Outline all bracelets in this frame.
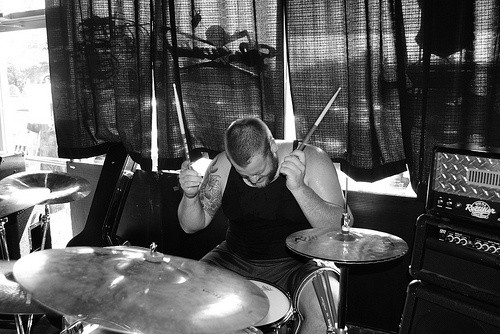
[184,192,198,199]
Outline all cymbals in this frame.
[0,259,42,314]
[285,227,409,265]
[0,187,50,218]
[11,246,269,334]
[0,170,92,204]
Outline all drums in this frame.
[245,276,295,330]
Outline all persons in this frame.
[178,118,354,334]
